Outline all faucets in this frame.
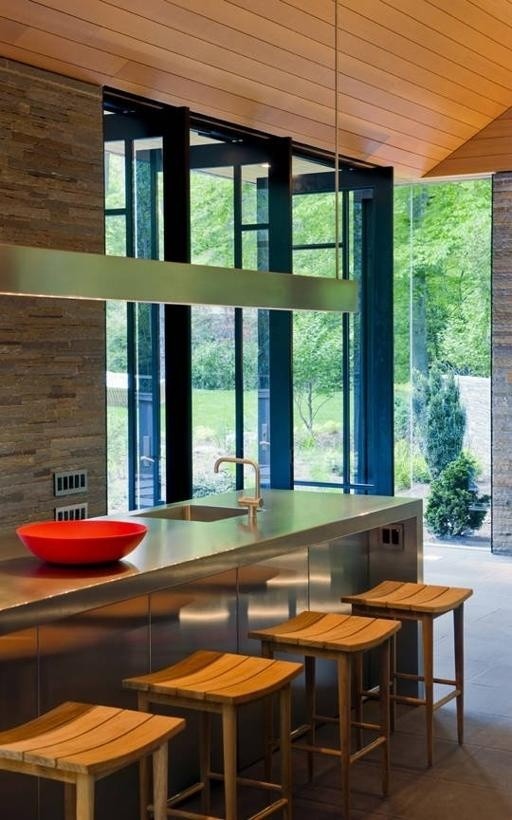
[213,455,263,518]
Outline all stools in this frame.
[0,701,187,820]
[122,649,305,820]
[340,580,472,767]
[247,611,393,820]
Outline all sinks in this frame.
[127,500,269,524]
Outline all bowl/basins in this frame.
[14,519,150,565]
